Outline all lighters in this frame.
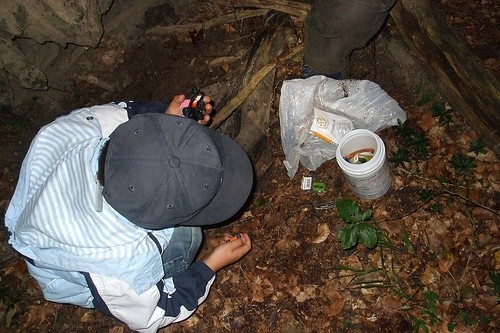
[230,234,242,241]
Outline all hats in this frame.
[103,112,253,230]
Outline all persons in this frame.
[302,0,396,79]
[4,95,251,333]
